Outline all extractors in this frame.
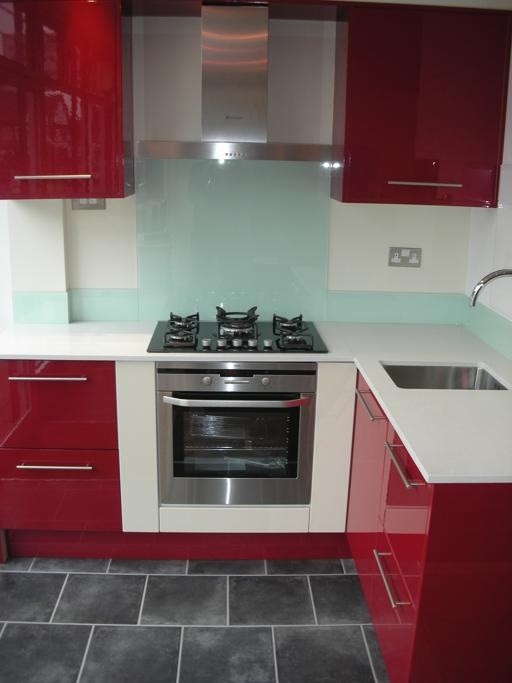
[138,4,347,165]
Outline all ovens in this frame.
[153,361,320,508]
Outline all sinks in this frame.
[377,359,512,390]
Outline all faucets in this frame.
[469,269,512,308]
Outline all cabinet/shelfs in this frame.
[0,360,122,533]
[368,421,512,683]
[331,1,512,207]
[0,0,135,199]
[345,371,389,607]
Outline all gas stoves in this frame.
[146,303,333,353]
